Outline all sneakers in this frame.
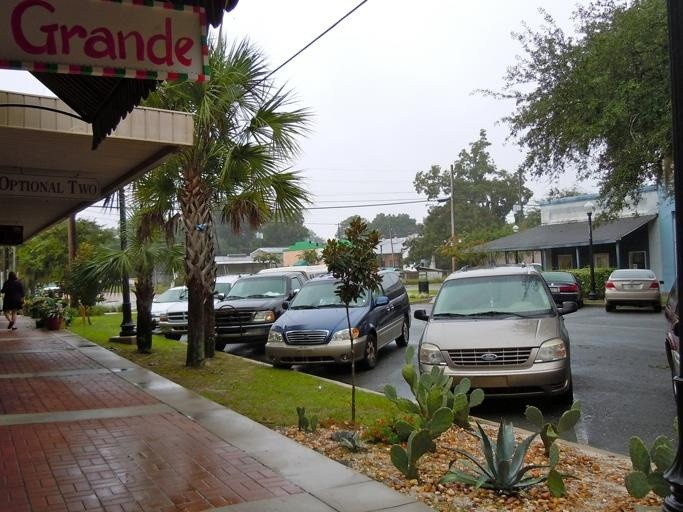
[8,321,17,330]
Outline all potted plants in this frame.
[42,300,64,330]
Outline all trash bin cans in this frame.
[419,282,428,294]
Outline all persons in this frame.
[0,271,25,329]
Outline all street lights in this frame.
[437,165,457,272]
[513,225,519,264]
[584,201,598,300]
